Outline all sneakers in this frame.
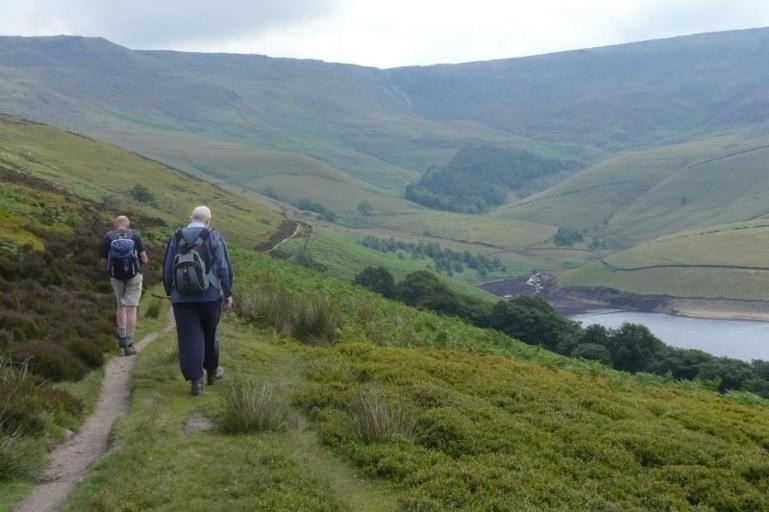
[207,367,224,384]
[190,379,203,395]
[119,337,137,356]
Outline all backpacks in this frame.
[171,227,213,294]
[107,231,139,281]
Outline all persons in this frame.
[99,215,149,356]
[162,205,234,396]
